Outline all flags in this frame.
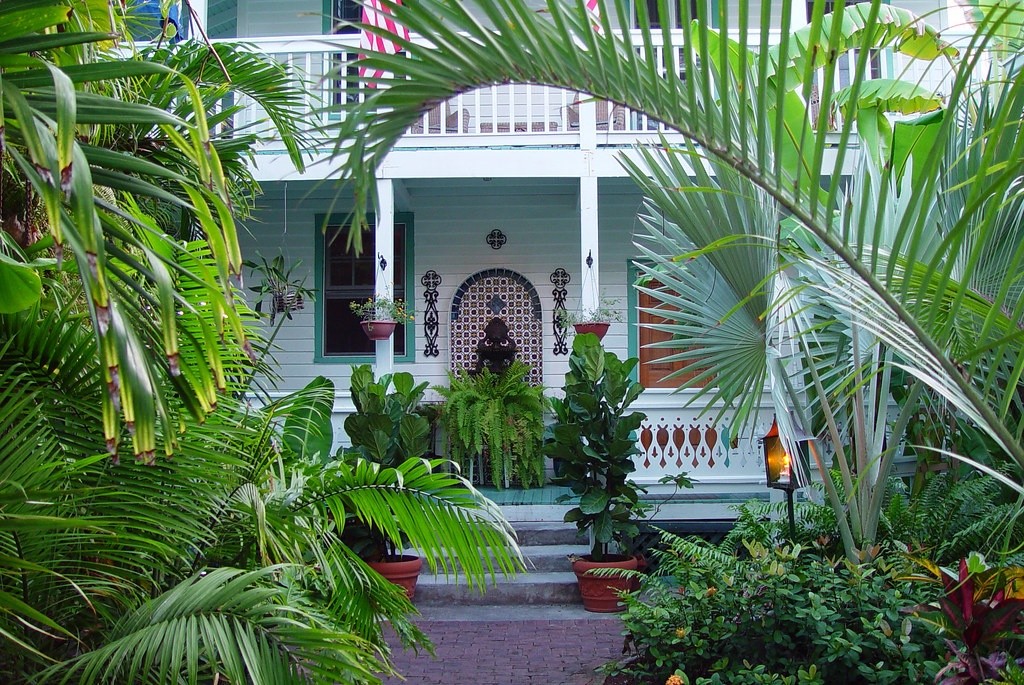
[587,0,602,35]
[157,0,184,50]
[358,0,410,88]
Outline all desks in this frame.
[480,122,558,133]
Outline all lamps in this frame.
[757,411,819,546]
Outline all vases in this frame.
[359,321,398,341]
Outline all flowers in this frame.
[349,281,420,332]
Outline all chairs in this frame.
[412,101,470,134]
[810,85,836,131]
[560,91,625,131]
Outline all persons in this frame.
[477,318,516,375]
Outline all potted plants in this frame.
[241,247,320,326]
[553,286,627,341]
[337,364,433,603]
[537,332,700,615]
